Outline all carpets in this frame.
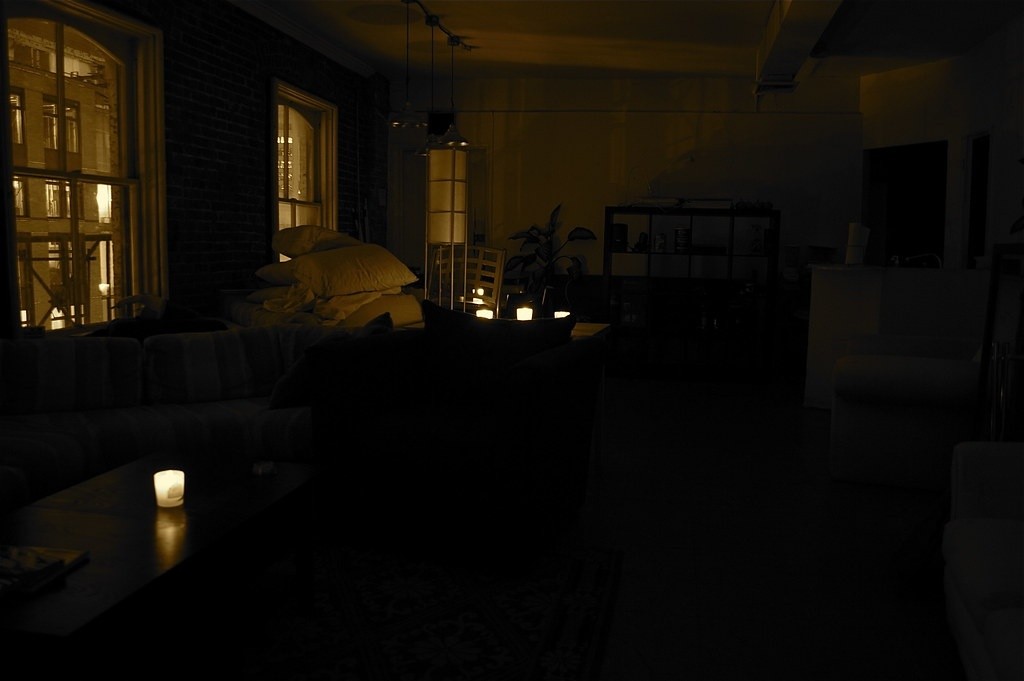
[225,537,624,681]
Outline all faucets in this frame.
[904,252,944,271]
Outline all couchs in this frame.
[303,329,609,579]
[829,331,982,489]
[0,322,312,513]
[942,440,1024,681]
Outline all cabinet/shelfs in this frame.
[803,264,1024,410]
[602,206,781,387]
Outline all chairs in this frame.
[427,245,507,319]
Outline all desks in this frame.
[0,447,317,681]
[571,322,612,479]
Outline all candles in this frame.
[517,307,533,320]
[476,309,493,319]
[554,311,570,318]
[153,469,185,508]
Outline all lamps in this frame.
[386,0,429,129]
[424,147,469,313]
[414,14,450,157]
[435,34,469,147]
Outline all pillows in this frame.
[266,330,353,411]
[420,300,578,414]
[0,337,142,415]
[285,244,419,299]
[272,225,365,258]
[143,324,290,404]
[246,286,288,304]
[254,262,299,286]
[354,313,394,338]
[336,293,423,327]
